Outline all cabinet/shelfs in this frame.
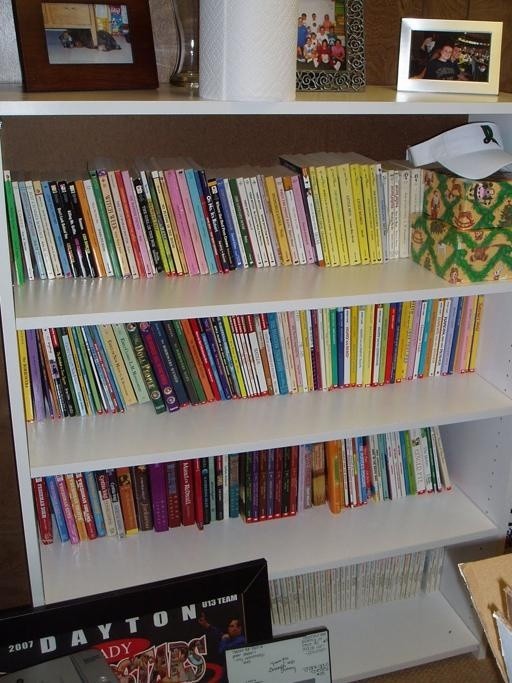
[0,0,510,681]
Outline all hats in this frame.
[404,122,511,181]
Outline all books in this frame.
[18,294,485,425]
[32,422,453,546]
[267,544,448,624]
[4,152,426,286]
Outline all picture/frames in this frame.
[10,0,161,94]
[395,19,503,96]
[295,0,367,93]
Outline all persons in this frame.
[298,12,345,70]
[196,612,245,658]
[409,36,489,82]
